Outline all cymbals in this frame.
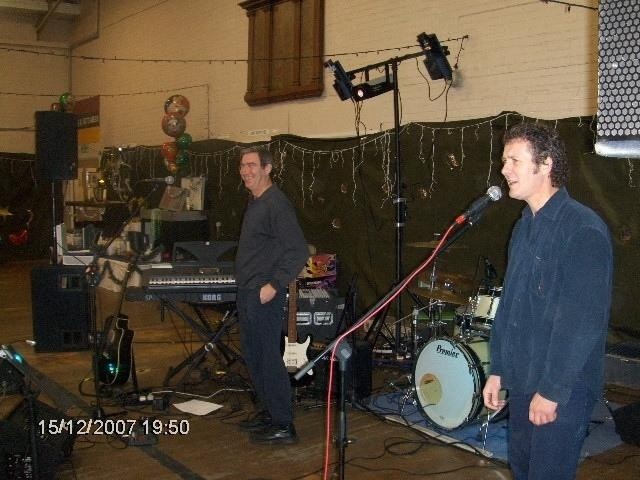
[404,241,469,250]
[406,269,479,306]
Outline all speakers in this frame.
[30,264,96,353]
[34,110,78,181]
[0,397,90,480]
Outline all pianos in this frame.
[64,199,127,208]
[141,261,289,303]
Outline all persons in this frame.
[231,144,311,445]
[481,122,613,480]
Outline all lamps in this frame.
[321,32,459,352]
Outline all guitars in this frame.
[92,246,145,387]
[281,278,314,388]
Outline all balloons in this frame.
[161,94,192,183]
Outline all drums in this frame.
[453,285,504,338]
[410,331,508,432]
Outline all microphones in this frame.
[457,186,503,225]
[138,176,175,185]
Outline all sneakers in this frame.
[239,410,273,432]
[249,421,297,442]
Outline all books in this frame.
[179,176,207,211]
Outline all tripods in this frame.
[346,51,448,354]
[72,185,162,446]
